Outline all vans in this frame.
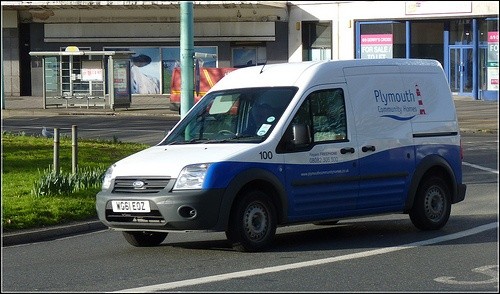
[94,58,467,252]
[168,67,238,114]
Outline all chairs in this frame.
[291,124,312,145]
[237,92,273,138]
